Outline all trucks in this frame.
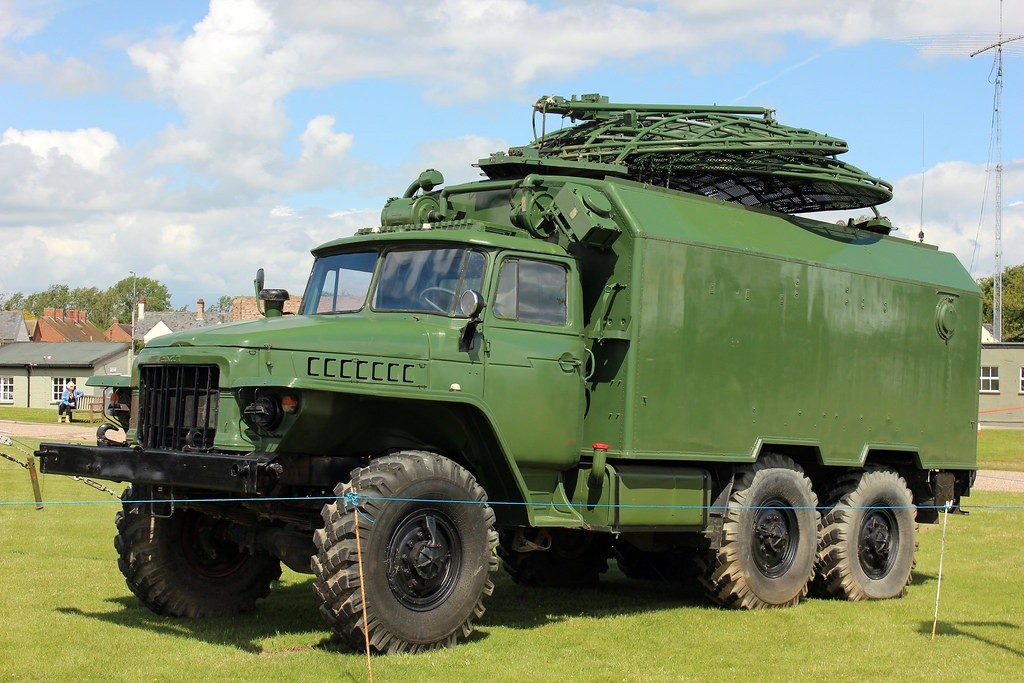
[32,95,985,655]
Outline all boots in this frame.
[65,415,70,424]
[58,415,62,423]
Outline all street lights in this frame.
[130,271,136,367]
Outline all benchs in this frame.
[51,395,105,424]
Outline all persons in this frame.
[57,381,84,423]
